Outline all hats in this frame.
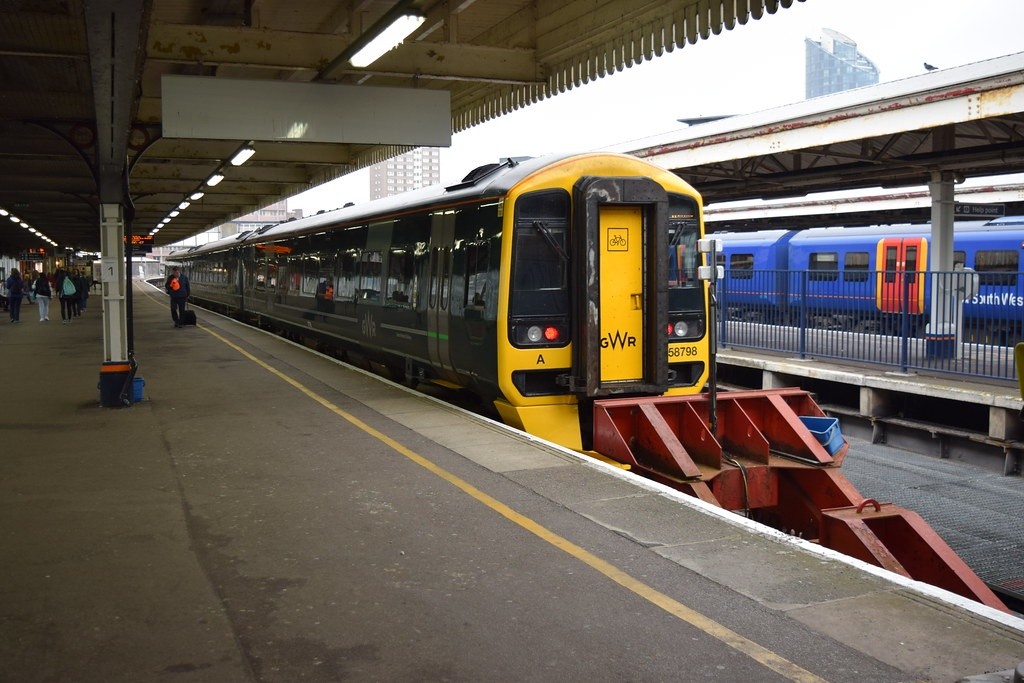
[172,266,179,271]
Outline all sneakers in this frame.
[40,318,45,322]
[45,316,50,321]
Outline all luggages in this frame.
[182,299,196,325]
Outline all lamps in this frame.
[149,189,206,236]
[230,146,256,166]
[206,170,225,187]
[0,208,59,247]
[347,7,428,67]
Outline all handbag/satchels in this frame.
[31,280,37,290]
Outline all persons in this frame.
[314,273,338,313]
[0,268,90,324]
[165,266,190,328]
[6,268,23,323]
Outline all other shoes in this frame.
[179,324,183,328]
[68,319,72,323]
[13,320,22,323]
[175,319,179,327]
[10,318,15,322]
[63,320,67,325]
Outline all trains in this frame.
[164,151,725,469]
[664,215,1024,347]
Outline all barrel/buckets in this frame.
[925,322,956,359]
[100,361,132,407]
[133,376,145,401]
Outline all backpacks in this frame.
[10,277,21,293]
[63,275,77,296]
